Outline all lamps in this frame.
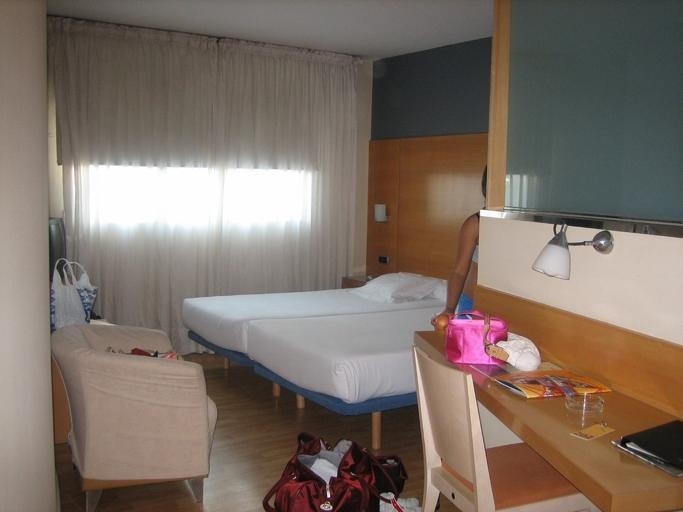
[531,218,614,281]
[373,203,387,223]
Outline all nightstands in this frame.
[341,271,387,289]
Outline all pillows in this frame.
[347,272,444,304]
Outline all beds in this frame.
[183,289,446,371]
[245,307,447,450]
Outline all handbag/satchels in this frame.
[446,311,507,368]
[260,431,409,511]
[483,314,541,373]
[49,256,99,330]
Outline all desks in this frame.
[413,329,683,512]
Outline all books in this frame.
[619,418,683,471]
[468,359,566,382]
[609,435,682,479]
[492,368,612,398]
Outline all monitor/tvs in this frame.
[49,216,68,282]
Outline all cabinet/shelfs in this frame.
[50,318,111,444]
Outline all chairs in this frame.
[50,324,218,512]
[411,344,593,512]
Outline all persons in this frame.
[430,165,487,331]
